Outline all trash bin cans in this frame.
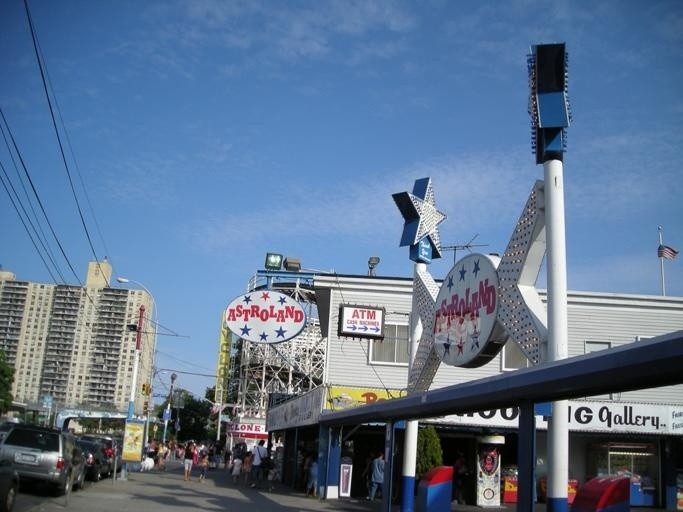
[570,475,630,512]
[418,466,454,512]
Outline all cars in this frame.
[0,421,122,495]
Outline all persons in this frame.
[368,451,386,503]
[141,435,320,498]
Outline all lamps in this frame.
[264,253,301,272]
[366,256,380,276]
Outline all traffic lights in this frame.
[142,384,146,396]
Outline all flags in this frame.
[657,245,679,262]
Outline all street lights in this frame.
[117,276,158,413]
[162,371,181,438]
